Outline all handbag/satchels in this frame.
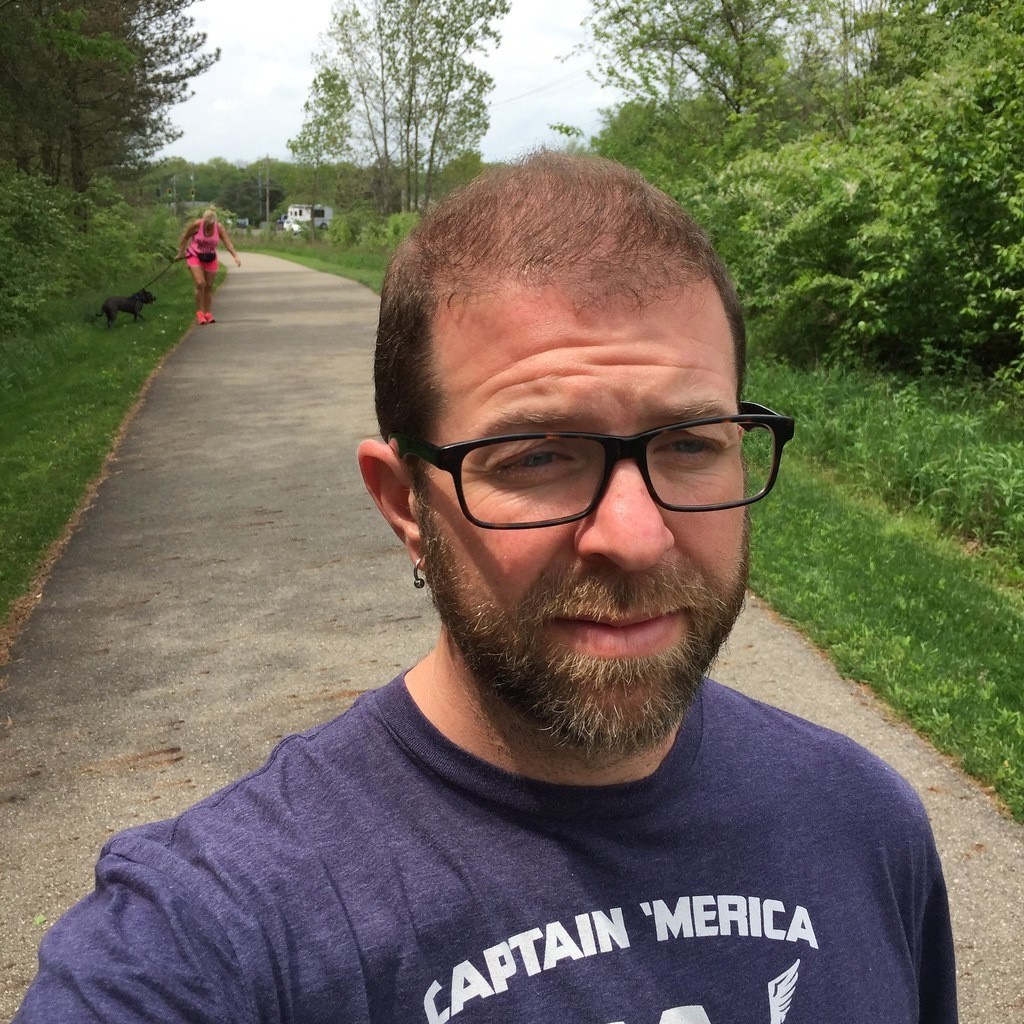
[190,246,216,262]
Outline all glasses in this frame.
[387,402,795,530]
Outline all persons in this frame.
[14,152,958,1024]
[175,206,241,325]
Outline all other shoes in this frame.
[196,311,207,325]
[204,312,215,323]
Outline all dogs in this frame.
[95,287,155,329]
